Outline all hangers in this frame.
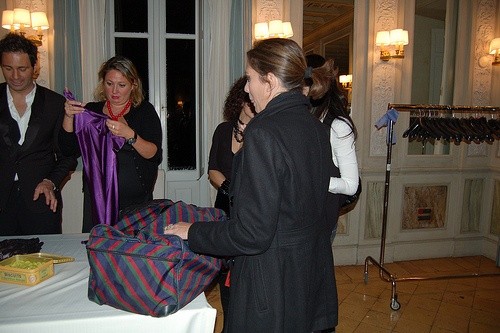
[402,104,500,146]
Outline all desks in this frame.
[0,233,218,333]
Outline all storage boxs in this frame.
[0,252,74,287]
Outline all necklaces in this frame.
[107,100,132,121]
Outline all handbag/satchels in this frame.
[83,198,232,317]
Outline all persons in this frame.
[60,55,161,235]
[162,38,362,333]
[206,75,256,216]
[301,51,359,197]
[0,34,81,235]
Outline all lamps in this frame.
[488,37,500,66]
[254,19,294,41]
[375,29,409,61]
[338,73,352,88]
[1,8,50,47]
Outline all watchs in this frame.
[127,131,139,146]
